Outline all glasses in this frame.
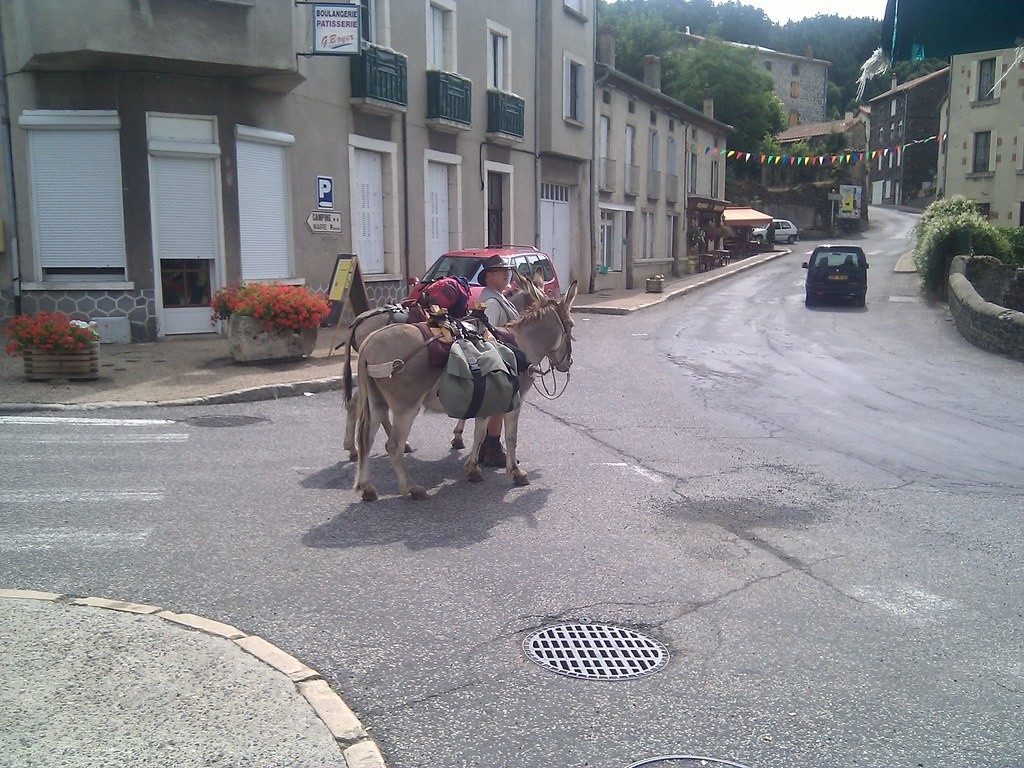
[486,266,512,275]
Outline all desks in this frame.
[698,254,713,270]
[716,250,729,265]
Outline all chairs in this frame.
[698,249,730,273]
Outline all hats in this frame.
[477,254,516,286]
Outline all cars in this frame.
[750,219,800,244]
[408,245,562,329]
[801,244,869,308]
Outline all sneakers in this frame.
[479,443,487,463]
[483,443,520,467]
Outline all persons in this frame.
[766,219,775,251]
[937,187,943,200]
[476,255,521,468]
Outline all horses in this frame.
[341,266,579,502]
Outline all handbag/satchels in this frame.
[439,339,522,419]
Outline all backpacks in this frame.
[417,276,471,317]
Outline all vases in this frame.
[23,344,99,380]
[222,315,318,364]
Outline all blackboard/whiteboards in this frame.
[319,252,372,325]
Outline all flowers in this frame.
[208,279,333,338]
[4,314,99,355]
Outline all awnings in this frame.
[722,207,774,227]
